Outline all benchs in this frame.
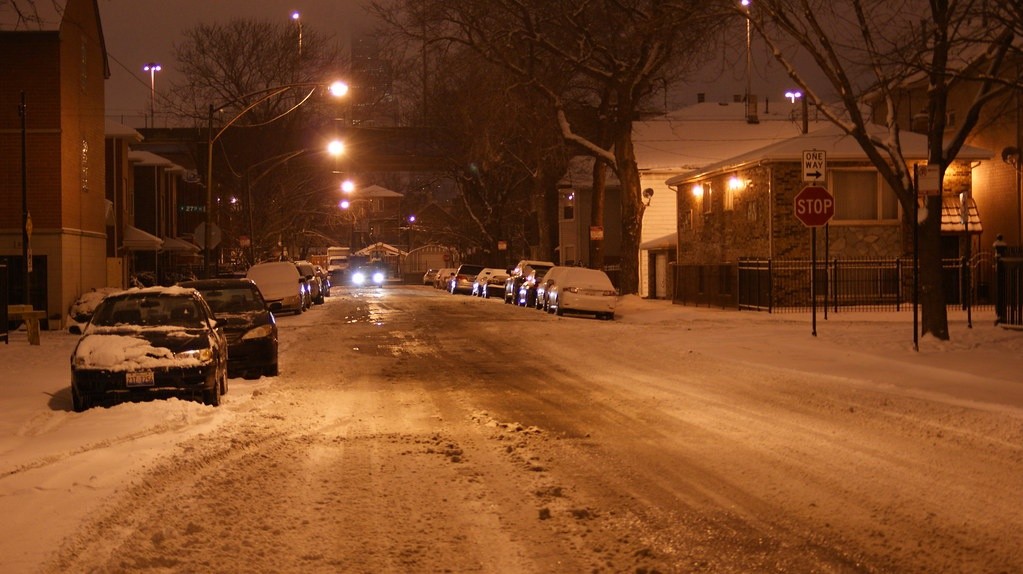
[7,311,47,345]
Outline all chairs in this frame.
[114,308,143,326]
[170,304,196,321]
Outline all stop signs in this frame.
[794,185,836,229]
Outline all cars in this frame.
[356,265,383,288]
[423,260,617,320]
[168,279,283,378]
[67,285,229,412]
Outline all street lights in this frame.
[144,61,162,127]
[292,9,306,93]
[245,139,345,266]
[203,81,349,277]
[282,178,354,257]
[741,0,753,101]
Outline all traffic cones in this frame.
[241,246,352,316]
